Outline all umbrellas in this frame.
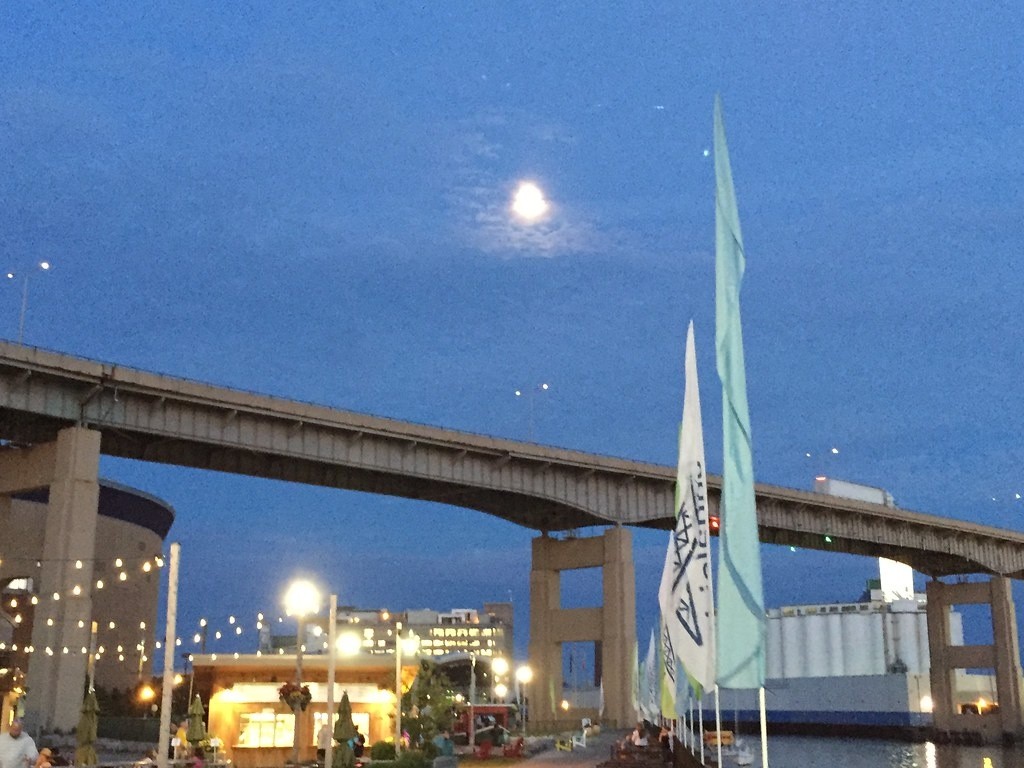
[77,689,99,766]
[332,690,356,768]
[186,695,205,758]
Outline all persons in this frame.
[483,715,490,727]
[623,735,633,759]
[631,723,651,765]
[659,727,680,768]
[317,725,334,761]
[0,723,68,768]
[354,726,365,757]
[403,728,409,751]
[168,722,188,759]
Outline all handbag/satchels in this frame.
[172,738,181,747]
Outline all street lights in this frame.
[516,666,532,737]
[280,578,322,767]
